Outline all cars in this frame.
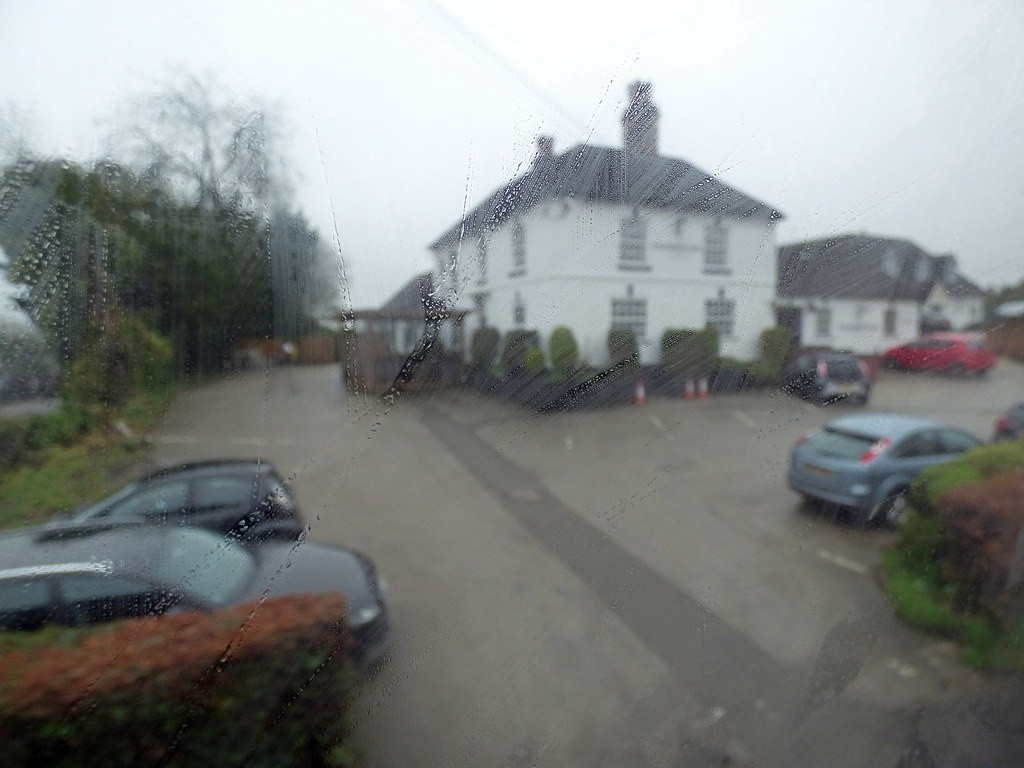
[70,459,307,541]
[782,349,872,404]
[990,401,1024,442]
[880,332,998,376]
[786,412,986,531]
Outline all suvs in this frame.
[0,516,390,670]
